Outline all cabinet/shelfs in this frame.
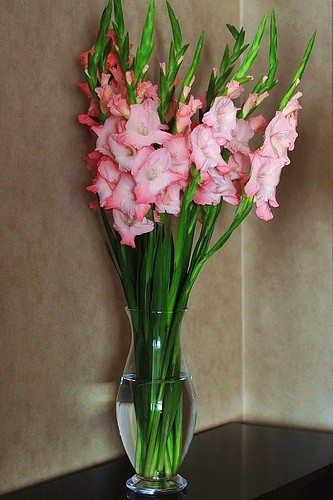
[1,422,332,498]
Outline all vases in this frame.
[115,306,198,494]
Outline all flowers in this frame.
[77,1,317,479]
